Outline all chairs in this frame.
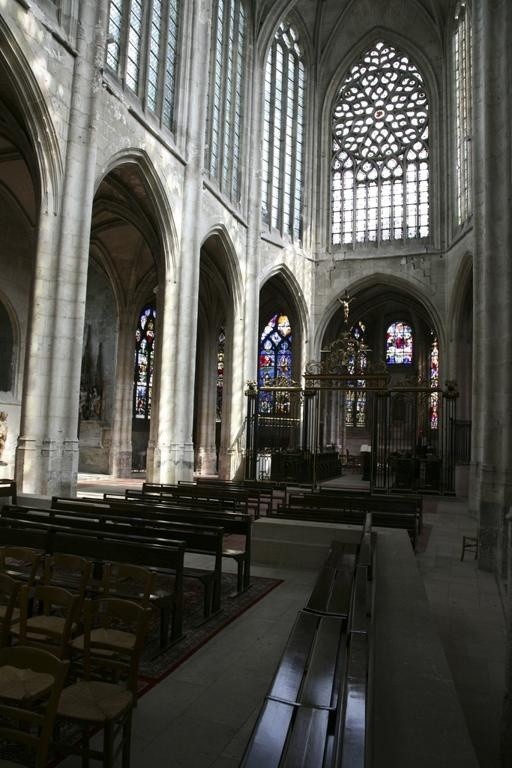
[1,584,151,768]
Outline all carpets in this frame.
[1,555,286,768]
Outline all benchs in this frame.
[0,477,424,660]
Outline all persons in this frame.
[336,296,357,323]
[85,385,103,420]
[0,410,10,467]
[78,384,90,422]
